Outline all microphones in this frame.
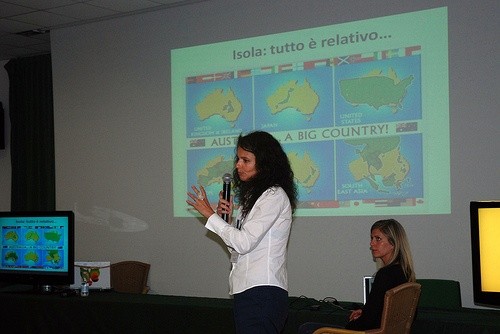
[222,173,231,223]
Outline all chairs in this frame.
[312,282,422,334]
[416,279,462,307]
[109,261,152,294]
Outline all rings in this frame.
[200,197,205,200]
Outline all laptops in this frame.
[363,277,377,306]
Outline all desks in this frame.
[0,286,500,334]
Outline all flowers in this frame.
[80,266,100,285]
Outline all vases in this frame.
[81,284,89,296]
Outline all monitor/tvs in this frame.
[470,201,500,309]
[0,211,74,296]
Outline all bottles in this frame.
[81,278,89,296]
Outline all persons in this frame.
[185,132,298,334]
[297,218,416,334]
